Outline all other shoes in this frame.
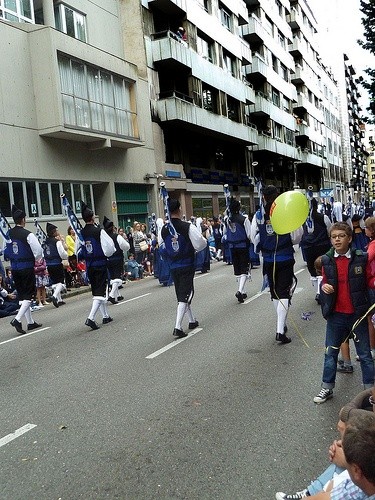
[235,291,247,303]
[85,318,100,330]
[108,295,123,304]
[102,316,113,324]
[50,296,59,308]
[27,321,43,331]
[276,333,292,343]
[58,301,66,306]
[173,328,188,337]
[30,301,50,312]
[336,360,354,373]
[10,318,26,334]
[189,321,199,329]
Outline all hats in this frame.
[46,222,58,232]
[81,201,94,219]
[12,204,25,221]
[167,194,181,211]
[103,216,113,229]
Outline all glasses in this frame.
[331,233,348,240]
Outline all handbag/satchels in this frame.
[139,240,149,252]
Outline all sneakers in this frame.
[275,489,310,500]
[314,388,333,403]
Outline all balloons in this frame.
[270,191,309,235]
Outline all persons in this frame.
[319,200,374,222]
[313,217,375,402]
[66,226,77,287]
[251,184,303,342]
[75,202,116,329]
[276,385,375,500]
[224,200,251,303]
[3,205,42,334]
[102,217,130,303]
[160,197,207,338]
[0,267,20,318]
[113,220,158,284]
[41,223,69,308]
[34,258,49,308]
[299,198,332,303]
[181,215,224,261]
